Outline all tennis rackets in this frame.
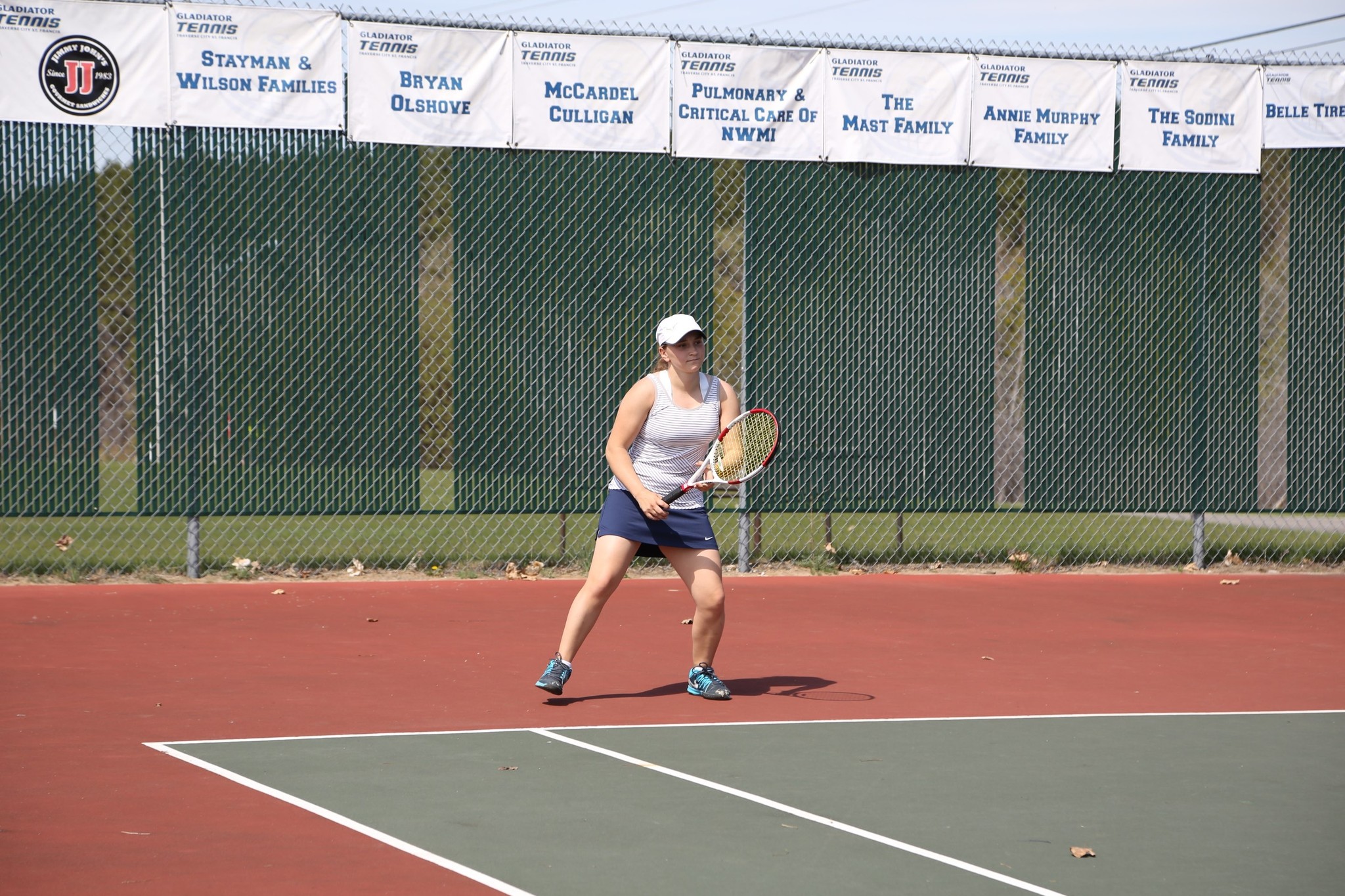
[659,407,782,510]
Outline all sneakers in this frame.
[687,662,731,699]
[535,652,572,695]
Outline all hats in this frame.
[655,314,707,347]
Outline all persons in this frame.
[535,313,745,698]
